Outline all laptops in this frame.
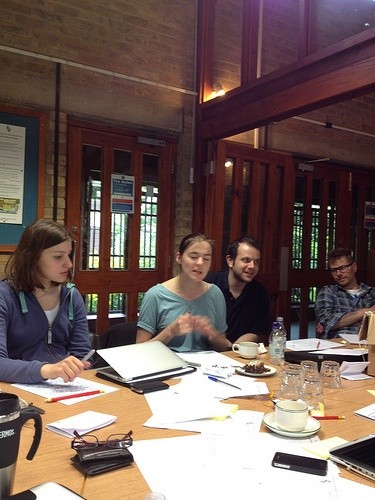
[96,340,188,382]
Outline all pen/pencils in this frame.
[314,416,345,420]
[317,342,321,348]
[45,390,105,403]
[208,377,241,390]
[186,361,202,367]
[82,350,95,362]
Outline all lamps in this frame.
[212,82,225,97]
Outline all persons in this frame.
[135,232,228,352]
[0,218,94,383]
[203,236,271,345]
[315,248,375,340]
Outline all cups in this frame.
[0,392,43,500]
[320,361,341,389]
[273,399,309,432]
[232,342,259,359]
[279,360,324,409]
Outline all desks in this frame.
[0,337,375,500]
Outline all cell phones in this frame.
[272,452,328,476]
[131,381,169,394]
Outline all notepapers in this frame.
[299,436,349,460]
[204,403,239,421]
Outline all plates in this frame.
[235,365,277,377]
[262,412,321,437]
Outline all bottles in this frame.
[268,317,287,365]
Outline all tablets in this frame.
[328,435,375,479]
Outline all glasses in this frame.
[329,262,354,275]
[71,430,133,450]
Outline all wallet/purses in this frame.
[72,439,134,475]
